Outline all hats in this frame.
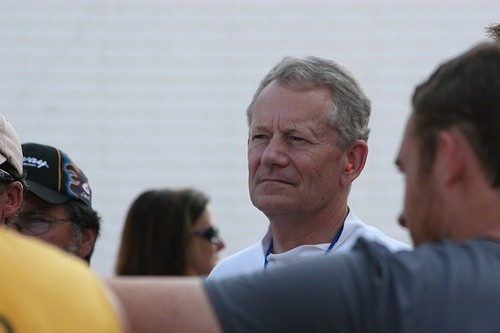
[20,142,92,207]
[0,114,23,175]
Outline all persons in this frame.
[207,56,412,281]
[0,114,123,333]
[100,40,500,333]
[5,142,99,267]
[115,188,226,278]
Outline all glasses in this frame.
[19,211,71,236]
[192,226,219,244]
